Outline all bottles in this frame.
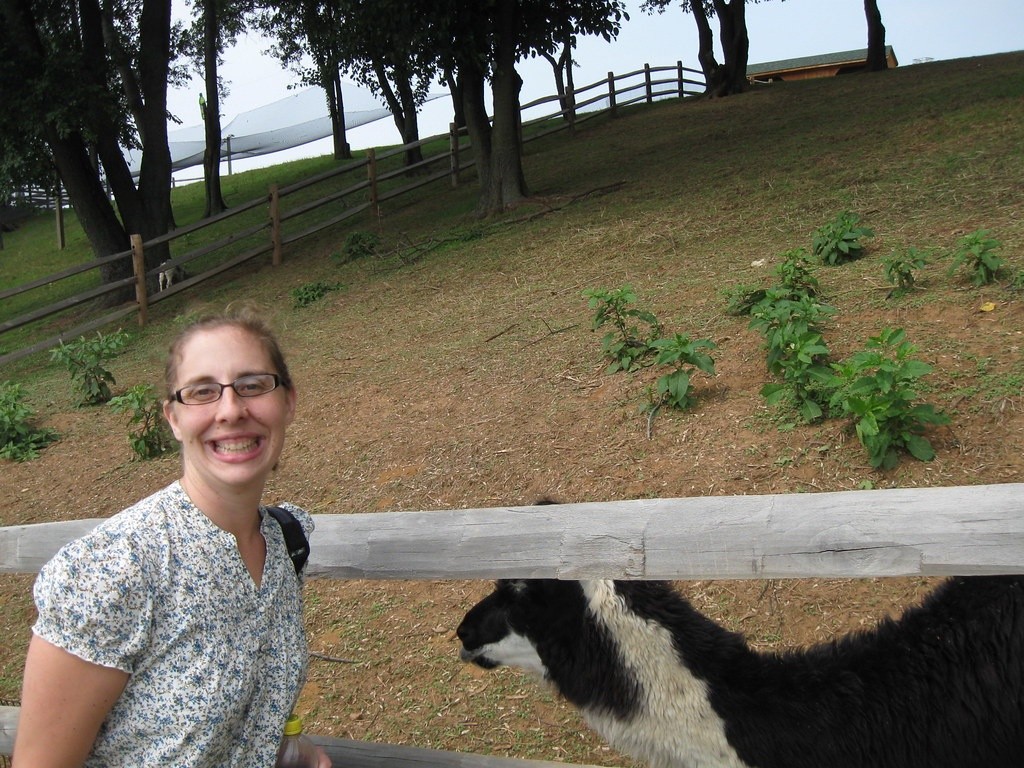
[275,714,319,768]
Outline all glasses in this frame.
[169,373,291,405]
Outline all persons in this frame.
[11,312,335,768]
